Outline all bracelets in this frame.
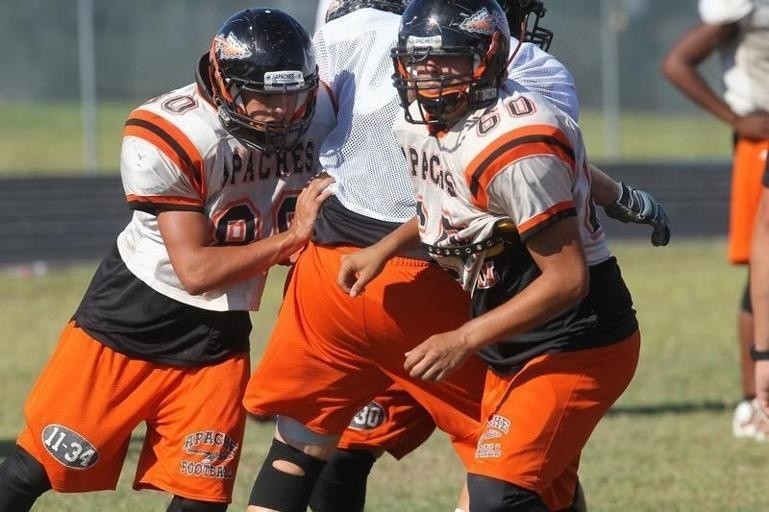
[749,342,769,362]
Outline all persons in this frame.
[2,7,335,511]
[745,144,769,425]
[242,0,673,510]
[658,0,769,440]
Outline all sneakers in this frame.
[733,400,768,443]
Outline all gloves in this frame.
[609,183,670,247]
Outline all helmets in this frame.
[327,1,552,125]
[210,7,319,154]
